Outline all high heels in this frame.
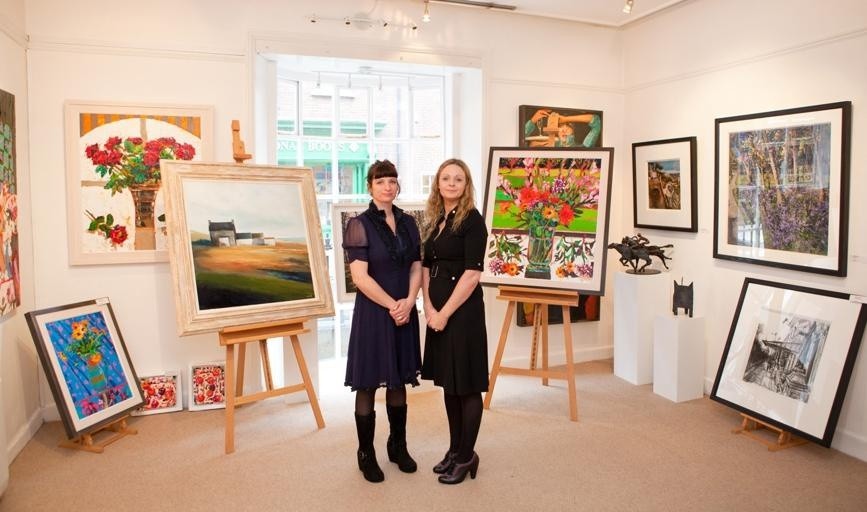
[438,449,479,484]
[432,448,459,473]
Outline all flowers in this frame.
[83,208,128,248]
[58,320,107,366]
[497,157,600,264]
[86,136,196,227]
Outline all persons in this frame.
[342,159,422,482]
[420,158,489,484]
[524,107,600,149]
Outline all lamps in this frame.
[422,1,430,22]
[622,0,633,15]
[309,14,417,30]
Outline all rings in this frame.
[398,316,402,320]
[434,329,438,332]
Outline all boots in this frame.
[386,404,417,473]
[355,410,384,483]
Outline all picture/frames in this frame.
[331,203,430,303]
[160,159,336,338]
[518,105,603,327]
[24,297,146,440]
[710,277,866,448]
[131,369,183,417]
[632,136,698,233]
[478,147,614,296]
[64,102,217,266]
[712,100,850,278]
[188,361,226,411]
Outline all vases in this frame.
[86,360,107,391]
[526,224,554,280]
[129,183,160,250]
